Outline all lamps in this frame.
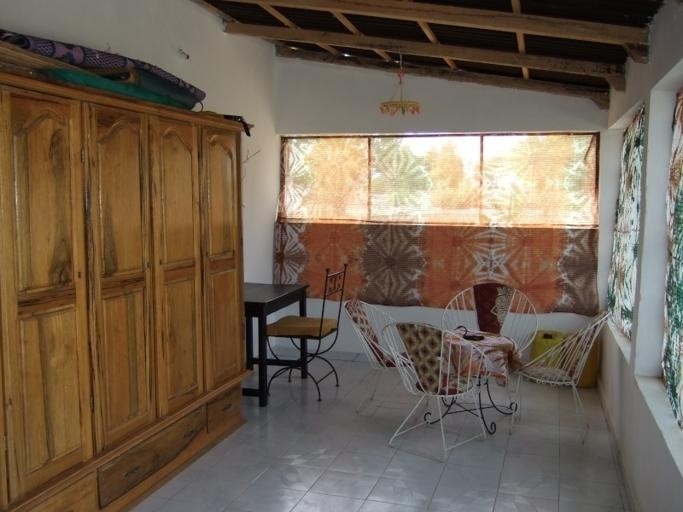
[380,52,421,115]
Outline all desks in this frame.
[243,284,309,407]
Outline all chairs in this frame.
[267,263,348,401]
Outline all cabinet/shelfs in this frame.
[0,73,254,512]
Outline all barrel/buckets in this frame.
[565,342,598,387]
[531,330,562,382]
